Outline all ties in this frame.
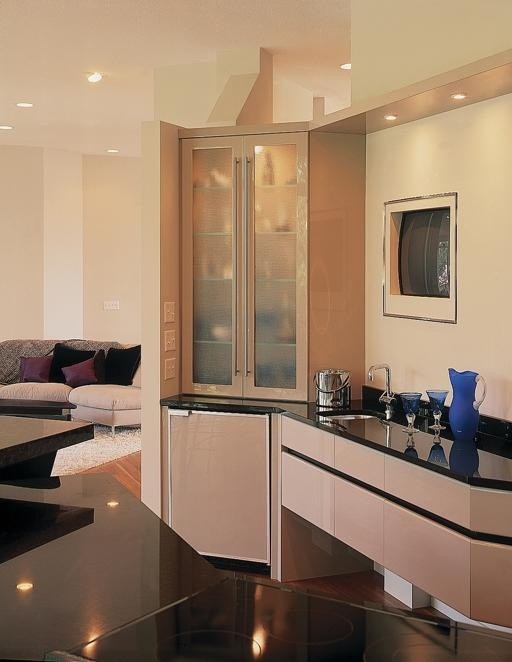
[383,195,456,323]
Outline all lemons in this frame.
[447,367,487,438]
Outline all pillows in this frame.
[18,341,141,387]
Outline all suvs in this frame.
[313,368,352,407]
[316,407,348,432]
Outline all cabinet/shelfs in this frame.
[166,408,272,564]
[280,412,362,556]
[178,131,312,404]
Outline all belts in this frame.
[426,430,449,468]
[399,392,422,433]
[426,390,448,430]
[402,432,420,460]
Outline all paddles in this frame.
[51,417,142,476]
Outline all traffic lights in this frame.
[18,341,141,387]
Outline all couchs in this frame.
[0,339,142,439]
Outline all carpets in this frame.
[51,417,142,476]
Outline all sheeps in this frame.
[385,410,395,449]
[368,362,397,410]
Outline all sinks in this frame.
[0,339,142,439]
